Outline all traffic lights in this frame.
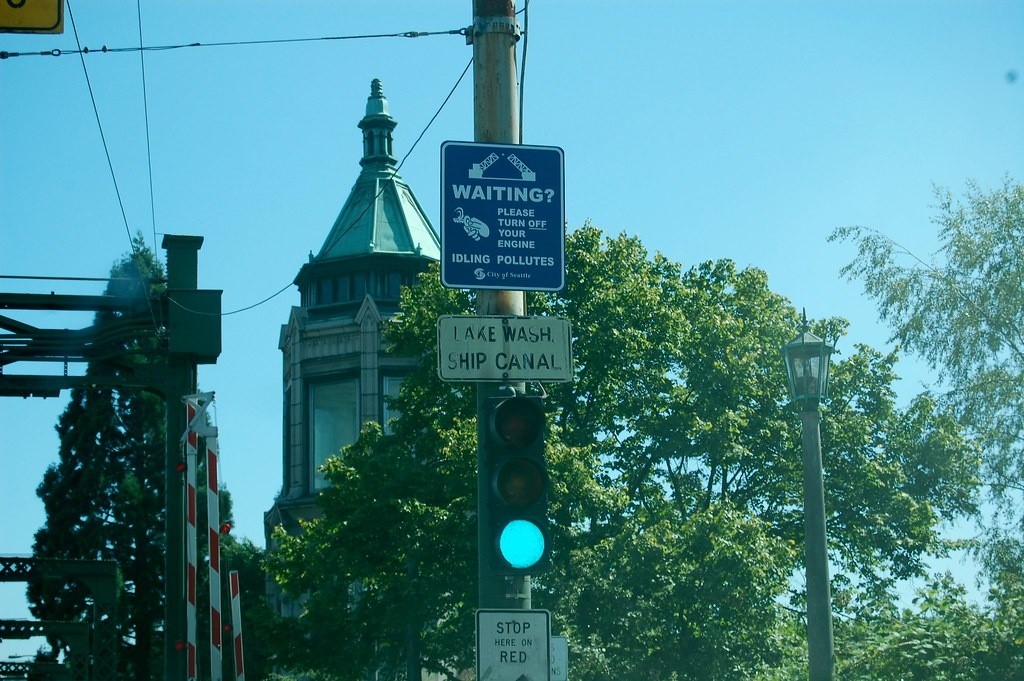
[489,396,551,571]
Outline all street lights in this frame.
[781,307,840,681]
